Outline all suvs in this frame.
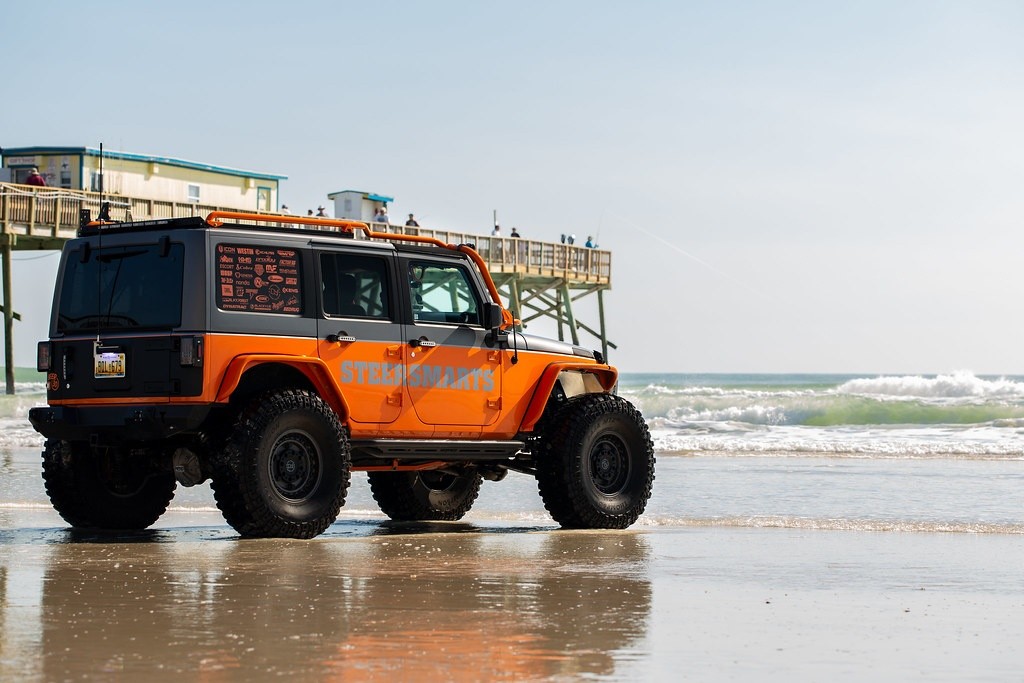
[28,144,656,539]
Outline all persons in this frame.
[305,208,315,230]
[510,227,521,260]
[279,204,291,227]
[316,205,331,231]
[491,224,504,261]
[24,166,46,187]
[585,235,598,273]
[405,213,419,235]
[372,205,393,241]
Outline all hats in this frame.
[28,168,39,175]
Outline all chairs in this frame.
[324,272,366,315]
